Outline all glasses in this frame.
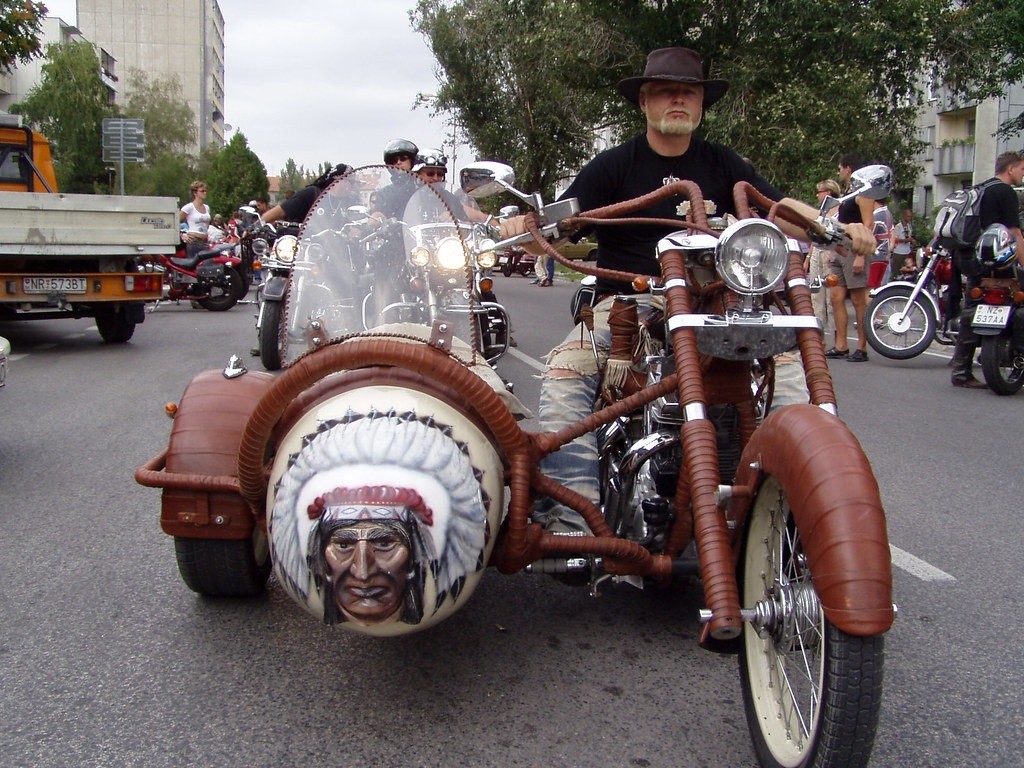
[197,190,207,192]
[392,156,410,164]
[816,190,826,194]
[837,167,845,172]
[423,155,447,165]
[421,170,444,177]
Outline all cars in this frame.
[555,239,598,261]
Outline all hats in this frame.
[617,48,730,109]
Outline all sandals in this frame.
[824,346,850,359]
[847,349,869,362]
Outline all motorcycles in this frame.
[862,187,1024,396]
[133,161,895,768]
[137,182,519,371]
[499,248,534,277]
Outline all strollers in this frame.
[891,244,928,284]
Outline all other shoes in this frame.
[530,279,554,287]
[250,347,260,355]
[954,378,987,389]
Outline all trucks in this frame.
[0,113,180,344]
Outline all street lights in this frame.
[421,94,455,181]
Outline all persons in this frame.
[499,47,877,587]
[803,155,874,360]
[241,163,353,356]
[873,197,918,306]
[348,139,518,347]
[532,253,553,288]
[180,180,296,309]
[951,152,1024,389]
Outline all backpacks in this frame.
[934,180,1003,248]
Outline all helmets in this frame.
[412,148,448,173]
[383,139,418,161]
[976,223,1017,270]
